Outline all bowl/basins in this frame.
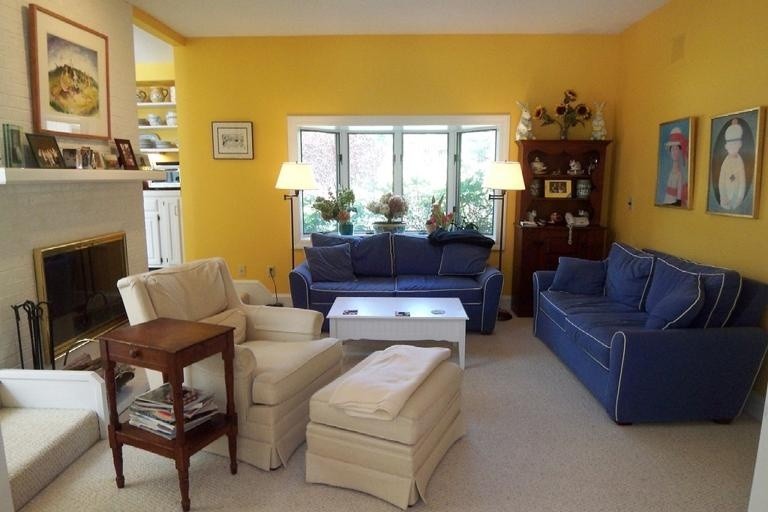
[148,114,161,125]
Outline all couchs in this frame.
[290,228,503,337]
[533,243,767,426]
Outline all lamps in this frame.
[480,160,526,322]
[274,161,320,272]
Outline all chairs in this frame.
[118,256,351,474]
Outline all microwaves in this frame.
[146,165,180,188]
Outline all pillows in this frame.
[642,273,706,331]
[647,257,726,325]
[604,240,653,312]
[301,244,357,282]
[437,227,494,277]
[549,251,605,300]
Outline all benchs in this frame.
[0,363,111,510]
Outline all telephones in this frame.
[565,212,590,228]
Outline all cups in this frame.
[166,111,177,124]
[169,87,175,102]
[82,150,96,170]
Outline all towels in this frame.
[330,342,455,420]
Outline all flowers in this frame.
[427,194,456,233]
[536,86,592,130]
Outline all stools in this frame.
[303,350,471,507]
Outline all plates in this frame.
[139,138,153,148]
[138,133,160,148]
[156,141,170,149]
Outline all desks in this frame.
[100,316,245,510]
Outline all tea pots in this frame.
[148,87,167,102]
[532,157,548,175]
[136,89,146,102]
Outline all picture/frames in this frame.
[114,137,141,169]
[24,132,70,169]
[703,106,767,219]
[542,178,572,198]
[28,3,113,142]
[211,120,255,160]
[654,115,697,211]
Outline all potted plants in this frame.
[315,182,357,235]
[365,192,409,233]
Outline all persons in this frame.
[719,119,746,209]
[38,141,60,167]
[552,182,565,193]
[665,127,688,206]
[12,133,22,164]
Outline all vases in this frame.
[424,221,437,233]
[560,125,569,138]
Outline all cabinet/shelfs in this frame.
[134,101,179,153]
[510,138,614,320]
[142,193,184,268]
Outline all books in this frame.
[129,382,218,439]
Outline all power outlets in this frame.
[265,265,276,277]
